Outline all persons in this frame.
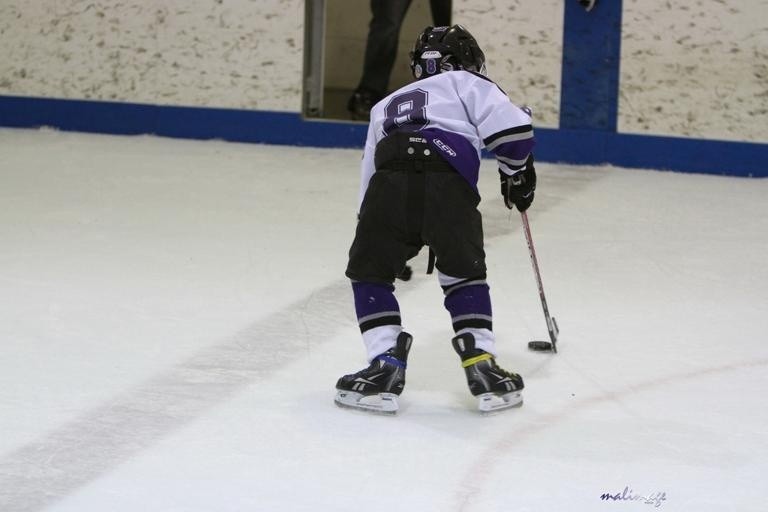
[347,0,452,118]
[335,22,542,397]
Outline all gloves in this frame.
[495,152,537,213]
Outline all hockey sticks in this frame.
[521,211,558,354]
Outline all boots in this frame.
[336,331,413,394]
[451,332,524,396]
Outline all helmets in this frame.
[408,24,486,81]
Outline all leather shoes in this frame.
[350,87,381,122]
